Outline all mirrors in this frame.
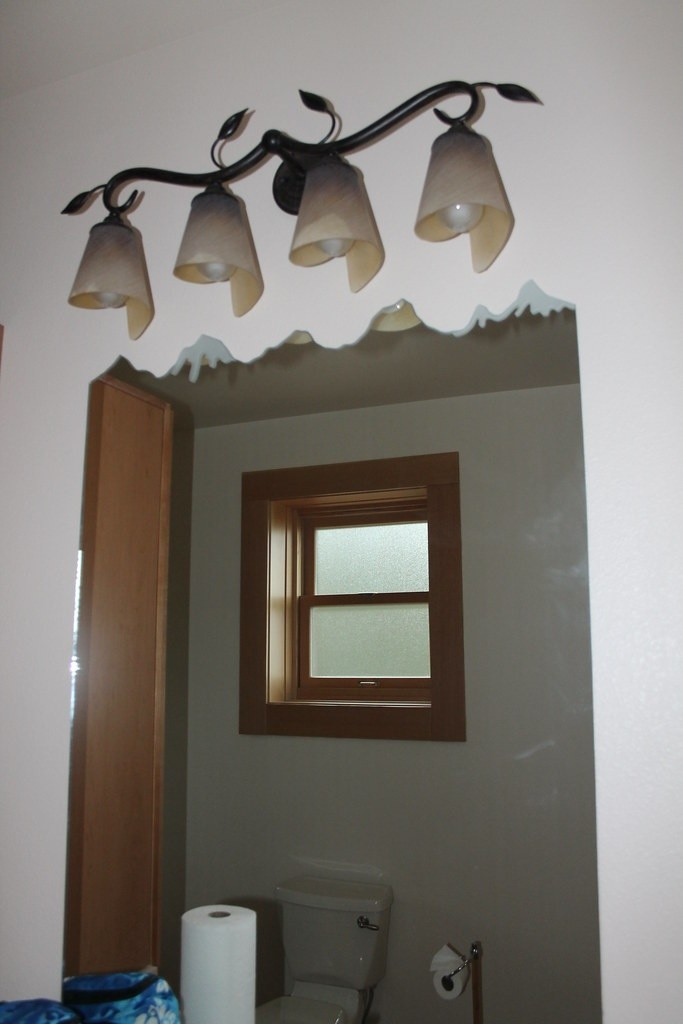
[61,298,606,1024]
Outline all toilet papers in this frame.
[180,904,257,1023]
[429,943,469,1000]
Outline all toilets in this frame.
[258,878,393,1024]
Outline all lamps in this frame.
[56,71,544,341]
